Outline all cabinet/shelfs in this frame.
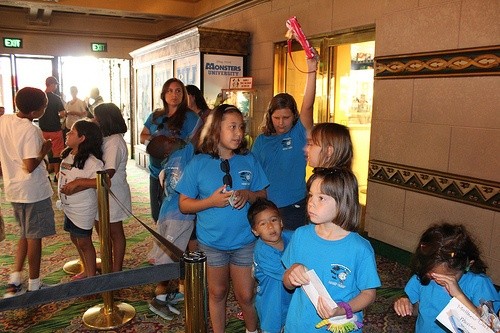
[132,28,253,170]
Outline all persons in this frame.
[247,47,320,232]
[243,122,253,150]
[0,86,55,297]
[247,199,298,333]
[58,119,105,301]
[303,122,353,173]
[58,86,105,148]
[392,222,500,333]
[0,107,4,115]
[140,78,213,321]
[54,104,131,274]
[179,103,268,333]
[283,167,378,333]
[38,77,66,184]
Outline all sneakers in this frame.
[149,292,176,320]
[149,295,180,315]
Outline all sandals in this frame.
[78,294,96,302]
[70,274,84,281]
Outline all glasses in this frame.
[421,245,466,259]
[312,167,344,181]
[221,159,232,189]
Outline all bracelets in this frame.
[338,301,353,319]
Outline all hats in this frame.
[46,77,60,85]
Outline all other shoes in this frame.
[4,284,24,298]
[173,293,184,301]
[54,176,58,182]
[25,284,48,292]
